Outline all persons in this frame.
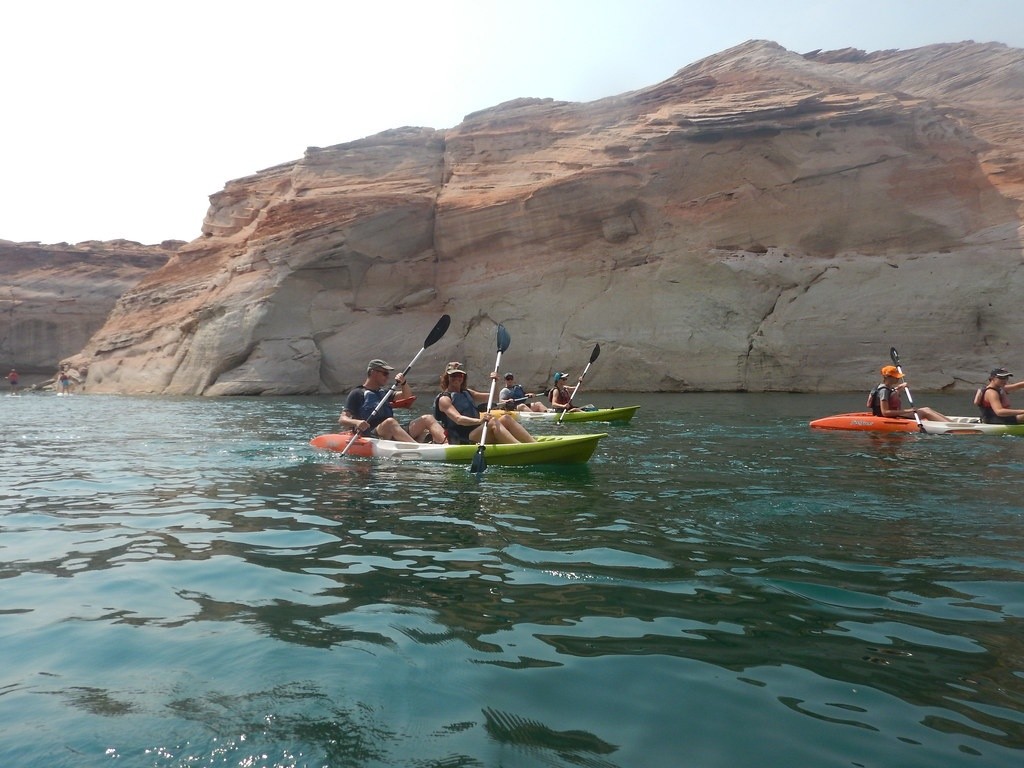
[433,361,538,444]
[5,369,24,396]
[339,359,448,444]
[872,365,953,422]
[552,372,588,412]
[60,371,71,395]
[498,372,553,412]
[979,368,1024,424]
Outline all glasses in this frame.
[450,373,463,377]
[996,377,1009,380]
[506,377,513,380]
[373,370,389,376]
[561,378,567,381]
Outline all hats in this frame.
[554,373,569,382]
[368,358,394,371]
[446,362,467,374]
[882,366,905,379]
[991,368,1014,377]
[504,372,513,379]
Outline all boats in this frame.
[478,404,642,427]
[808,409,1024,437]
[309,431,610,467]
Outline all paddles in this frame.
[477,388,552,413]
[555,341,601,426]
[889,346,928,434]
[468,323,511,474]
[338,314,452,459]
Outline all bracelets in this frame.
[401,380,407,386]
